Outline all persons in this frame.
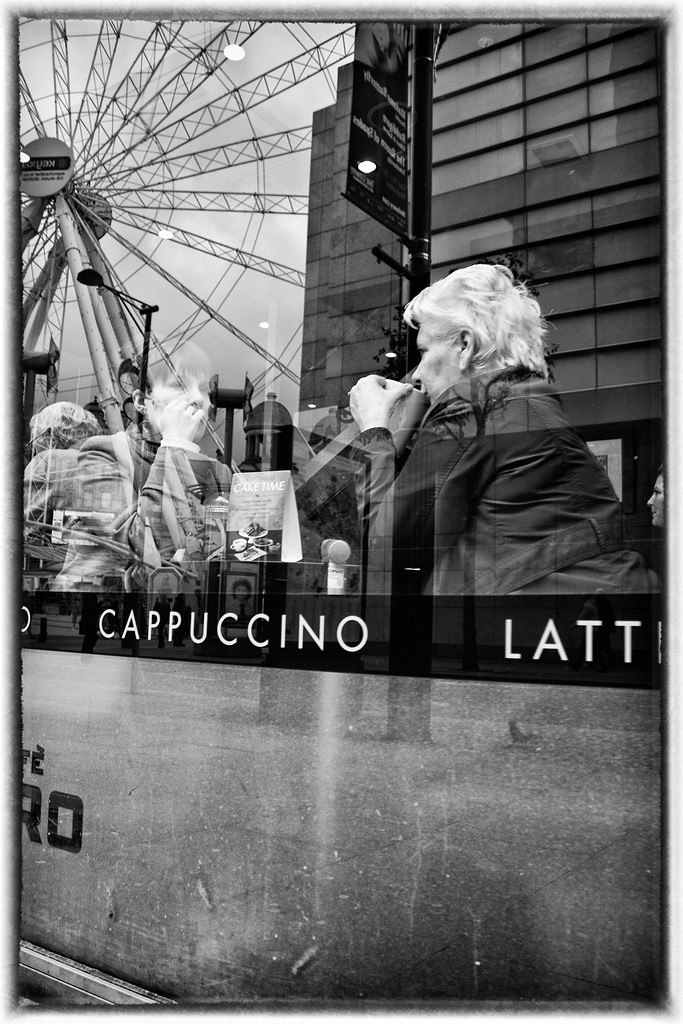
[22,402,103,564]
[49,338,232,592]
[347,263,667,594]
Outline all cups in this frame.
[202,492,231,557]
[383,378,426,453]
